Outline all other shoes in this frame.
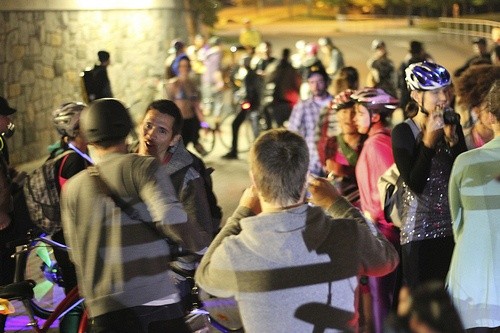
[222,150,237,160]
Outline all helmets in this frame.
[317,38,331,45]
[349,87,400,117]
[332,89,357,112]
[405,60,450,91]
[257,42,271,52]
[80,96,132,143]
[306,45,318,55]
[51,100,86,137]
[372,39,384,49]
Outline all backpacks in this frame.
[82,69,95,95]
[24,149,77,236]
[169,150,223,236]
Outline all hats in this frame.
[473,38,487,46]
[410,41,422,52]
[307,65,325,79]
[0,96,17,116]
[98,51,109,61]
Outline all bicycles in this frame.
[189,100,255,158]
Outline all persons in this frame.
[0,97,26,246]
[126,99,214,303]
[164,20,500,242]
[391,60,468,332]
[51,102,94,333]
[60,99,196,333]
[193,129,401,333]
[444,81,500,333]
[86,51,113,100]
